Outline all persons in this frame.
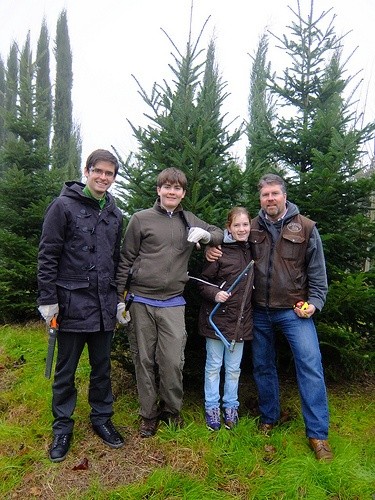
[38,149,336,462]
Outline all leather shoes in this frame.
[309,437,333,460]
[258,423,273,436]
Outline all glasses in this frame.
[89,168,113,177]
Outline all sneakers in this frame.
[205,407,221,431]
[159,411,183,428]
[49,433,73,462]
[140,415,158,437]
[93,419,125,448]
[223,407,238,429]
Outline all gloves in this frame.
[116,303,130,327]
[38,303,59,324]
[187,227,210,244]
